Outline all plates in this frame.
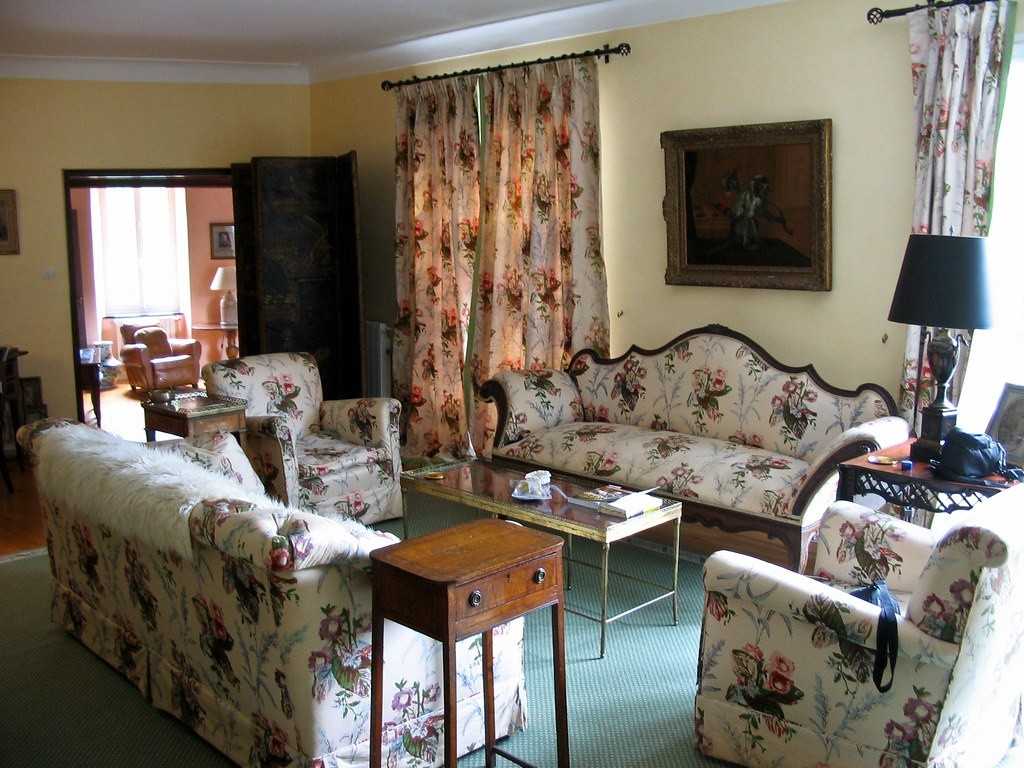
[866,455,899,464]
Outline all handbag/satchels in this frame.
[941,428,1001,478]
[849,580,900,614]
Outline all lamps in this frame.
[888,235,993,462]
[210,266,238,325]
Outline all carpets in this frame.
[0,459,738,768]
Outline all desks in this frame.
[193,324,240,361]
[400,458,682,658]
[142,390,251,464]
[835,436,1024,536]
[370,518,569,768]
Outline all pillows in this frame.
[133,327,173,358]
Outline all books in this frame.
[568,486,663,518]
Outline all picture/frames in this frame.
[210,223,236,259]
[0,189,20,254]
[661,118,833,291]
[985,382,1024,472]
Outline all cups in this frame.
[80,349,94,363]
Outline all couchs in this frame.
[15,416,526,768]
[120,323,201,392]
[201,352,403,525]
[694,482,1024,768]
[479,325,907,574]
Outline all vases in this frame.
[91,340,120,389]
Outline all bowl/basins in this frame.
[148,390,175,403]
